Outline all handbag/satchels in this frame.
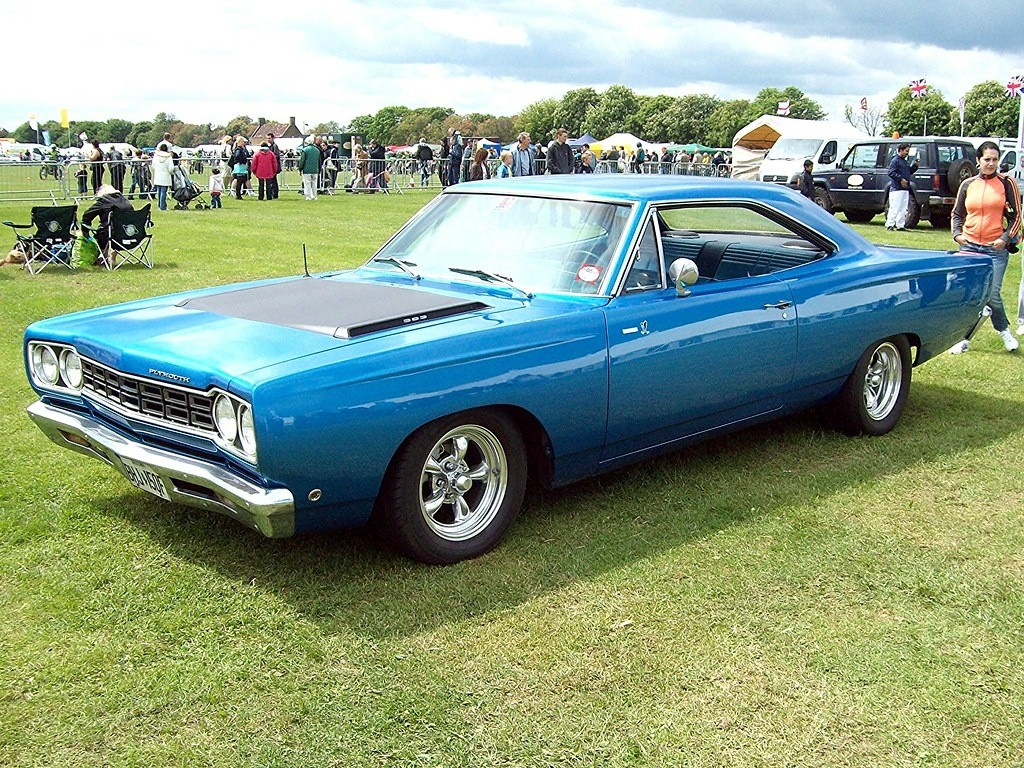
[221,145,229,162]
[233,163,248,174]
[327,148,338,171]
[356,163,365,171]
[1004,206,1020,254]
[383,172,391,183]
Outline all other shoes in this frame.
[298,190,335,195]
[128,193,157,200]
[305,195,317,201]
[418,188,428,191]
[364,190,374,194]
[234,189,259,200]
[158,207,170,212]
[382,189,389,194]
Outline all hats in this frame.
[95,184,122,201]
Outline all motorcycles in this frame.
[38,154,64,181]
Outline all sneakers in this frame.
[897,228,908,231]
[948,339,969,354]
[887,226,894,231]
[1016,324,1024,337]
[998,328,1019,353]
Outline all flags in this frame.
[776,98,790,115]
[909,79,926,98]
[60,108,69,128]
[29,114,37,130]
[861,97,867,110]
[1004,75,1024,97]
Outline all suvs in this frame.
[790,136,979,230]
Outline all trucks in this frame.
[993,147,1024,206]
[758,129,1003,196]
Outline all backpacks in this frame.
[419,146,430,161]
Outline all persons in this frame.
[949,140,1022,355]
[81,184,134,264]
[1,127,732,210]
[885,143,920,231]
[800,160,816,203]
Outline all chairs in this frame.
[83,204,154,270]
[2,205,77,277]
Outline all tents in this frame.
[730,114,870,180]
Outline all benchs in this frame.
[630,235,824,287]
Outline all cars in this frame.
[23,168,994,568]
[1,137,411,162]
